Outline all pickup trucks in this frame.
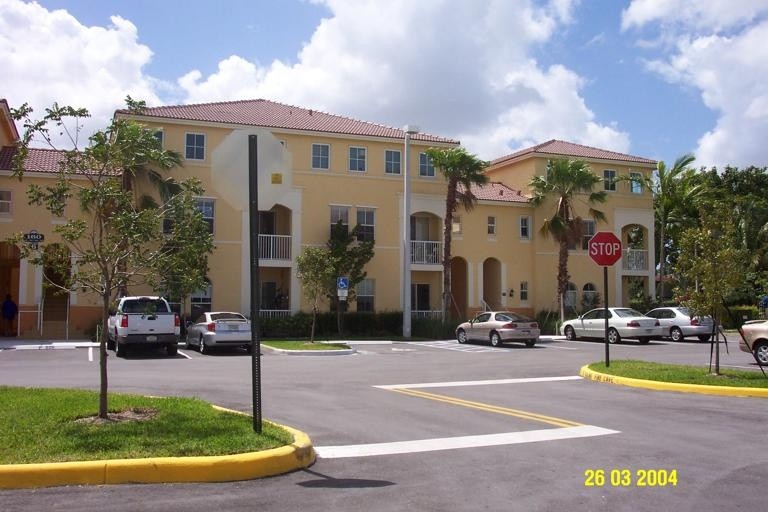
[104,294,182,356]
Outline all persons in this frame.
[1,293,18,337]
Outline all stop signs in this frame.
[588,232,621,267]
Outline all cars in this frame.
[737,318,768,367]
[641,307,724,344]
[185,309,251,353]
[560,306,663,346]
[454,311,541,349]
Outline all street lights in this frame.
[397,122,421,337]
